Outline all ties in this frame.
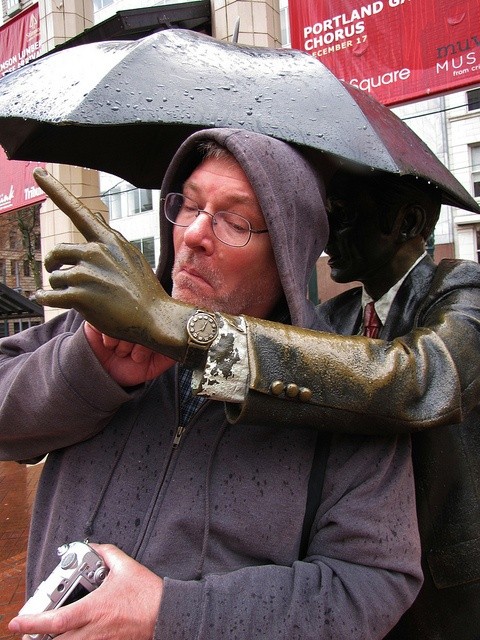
[361,303,380,338]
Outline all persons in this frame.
[0,127,423,639]
[31,165,480,639]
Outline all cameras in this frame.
[17,540,113,636]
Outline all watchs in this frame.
[183,307,220,370]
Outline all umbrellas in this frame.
[0,18,480,216]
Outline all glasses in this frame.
[159,192,267,247]
